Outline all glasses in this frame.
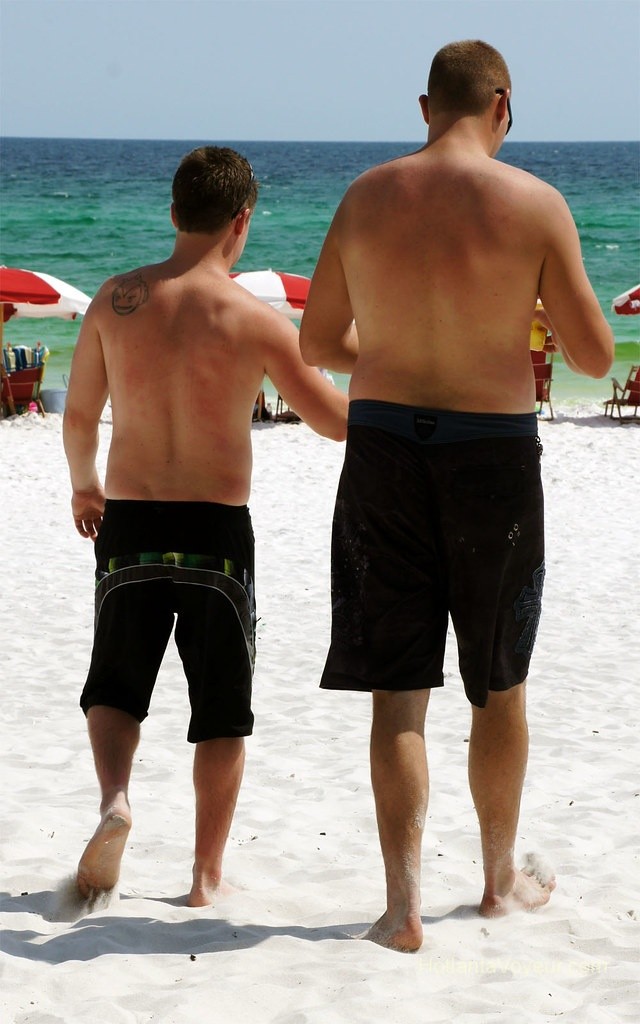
[495,87,512,135]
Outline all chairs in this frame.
[1,364,45,416]
[529,365,556,422]
[604,366,640,422]
[273,395,300,422]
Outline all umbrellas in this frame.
[229,270,312,319]
[612,283,640,315]
[0,264,96,367]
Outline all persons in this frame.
[297,42,613,950]
[60,146,348,909]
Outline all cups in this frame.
[529,295,550,351]
